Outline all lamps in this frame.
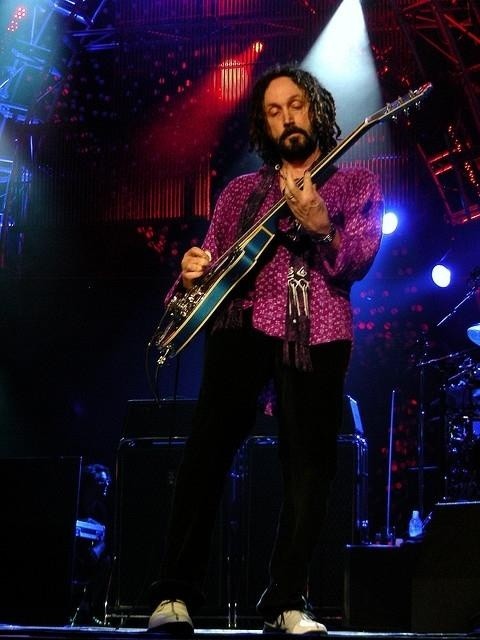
[430,245,471,289]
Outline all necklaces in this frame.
[278,171,301,181]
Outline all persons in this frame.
[81,464,112,497]
[143,66,384,637]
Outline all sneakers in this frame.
[142,593,195,635]
[259,607,332,639]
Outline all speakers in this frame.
[350,500,480,635]
[0,457,83,621]
[106,394,369,625]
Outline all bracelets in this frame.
[312,225,336,244]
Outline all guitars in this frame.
[154,85,429,357]
